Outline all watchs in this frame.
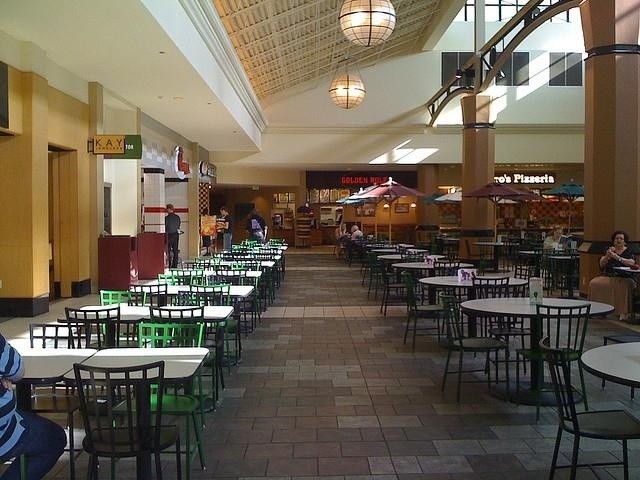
[618,256,622,263]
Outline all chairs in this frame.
[516,298,591,419]
[482,286,544,394]
[70,358,182,478]
[339,229,639,355]
[112,321,207,476]
[543,358,637,480]
[58,238,287,398]
[30,323,99,467]
[602,333,638,399]
[440,293,509,401]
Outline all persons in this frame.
[350,224,364,240]
[247,208,268,243]
[587,230,636,321]
[0,333,67,480]
[335,223,346,260]
[543,225,563,288]
[165,204,181,268]
[216,206,233,252]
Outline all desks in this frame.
[581,339,638,394]
[8,349,96,480]
[461,297,615,406]
[63,345,210,479]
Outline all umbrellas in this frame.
[348,177,427,245]
[462,182,546,242]
[336,187,380,233]
[421,192,445,203]
[434,191,462,202]
[540,182,584,233]
[503,188,544,237]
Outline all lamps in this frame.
[328,58,365,109]
[338,0,397,47]
